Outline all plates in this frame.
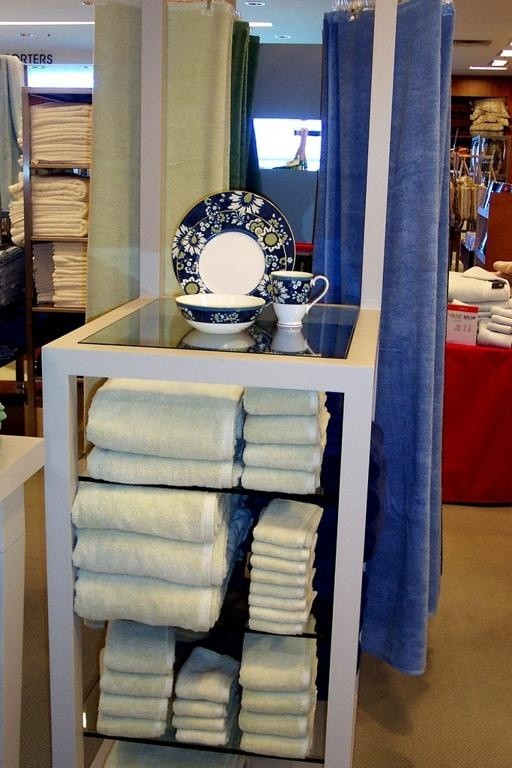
[170,189,296,306]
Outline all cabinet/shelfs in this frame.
[20,85,94,436]
[38,294,380,768]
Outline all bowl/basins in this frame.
[176,293,266,335]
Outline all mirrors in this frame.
[227,0,338,253]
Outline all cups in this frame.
[268,268,329,330]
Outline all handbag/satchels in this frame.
[450,177,487,222]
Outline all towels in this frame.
[247,499,323,638]
[238,613,318,760]
[82,374,332,493]
[447,267,512,348]
[97,619,177,740]
[6,99,87,309]
[71,479,253,635]
[170,644,238,746]
